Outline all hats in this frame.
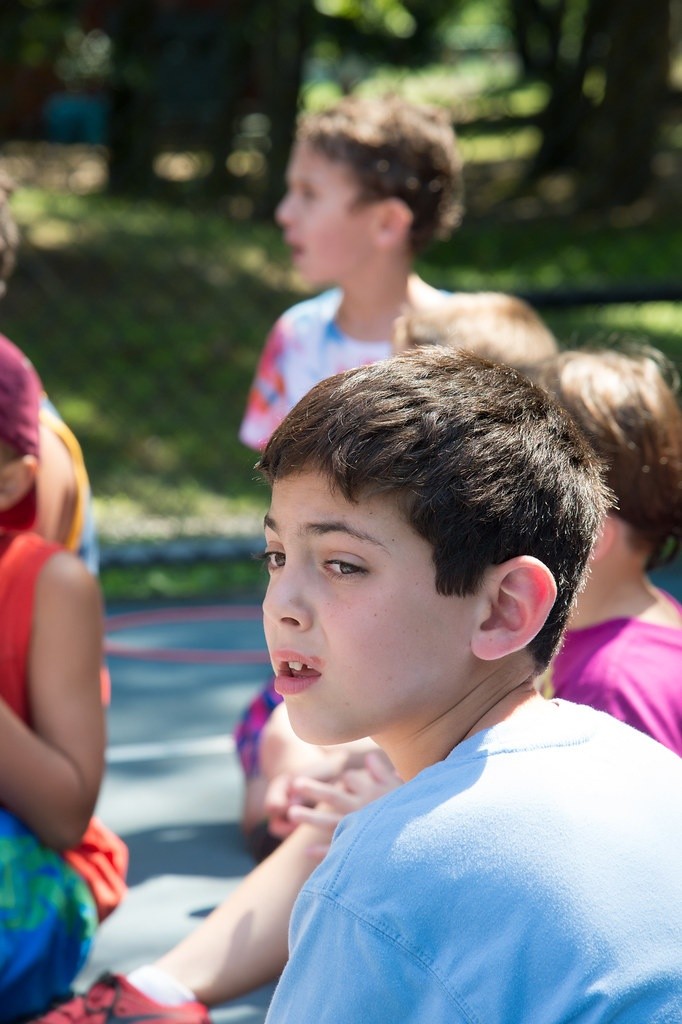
[1,334,39,531]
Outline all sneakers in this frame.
[23,969,208,1024]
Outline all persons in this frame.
[255,342,680,1023]
[35,94,681,1024]
[0,170,128,1024]
